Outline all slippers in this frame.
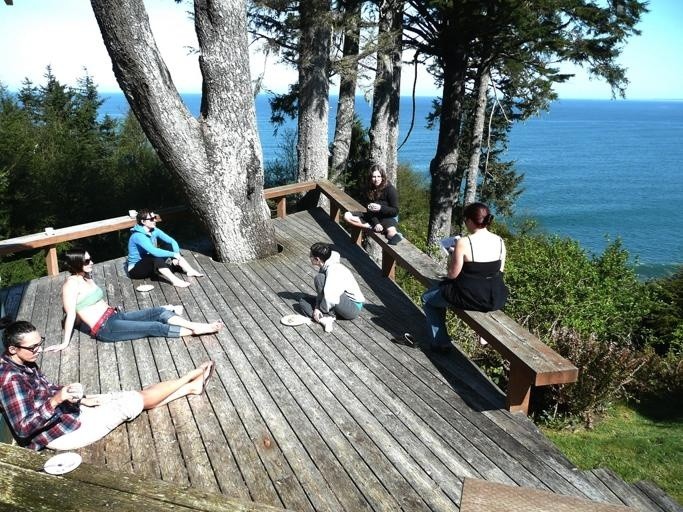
[199,362,214,394]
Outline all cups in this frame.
[368,203,377,211]
[66,382,85,405]
[107,284,114,295]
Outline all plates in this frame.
[136,284,155,292]
[280,315,308,326]
[43,452,83,476]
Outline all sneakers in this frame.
[391,331,420,348]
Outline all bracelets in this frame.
[53,394,63,404]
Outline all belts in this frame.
[89,306,116,337]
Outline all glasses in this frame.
[11,336,46,352]
[141,216,160,222]
[83,258,93,265]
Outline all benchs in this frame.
[455,472,638,511]
[0,175,316,281]
[316,178,582,421]
[0,438,294,512]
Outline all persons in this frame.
[344,165,403,246]
[421,203,506,353]
[1,321,215,449]
[299,243,365,332]
[44,249,220,352]
[126,210,203,287]
